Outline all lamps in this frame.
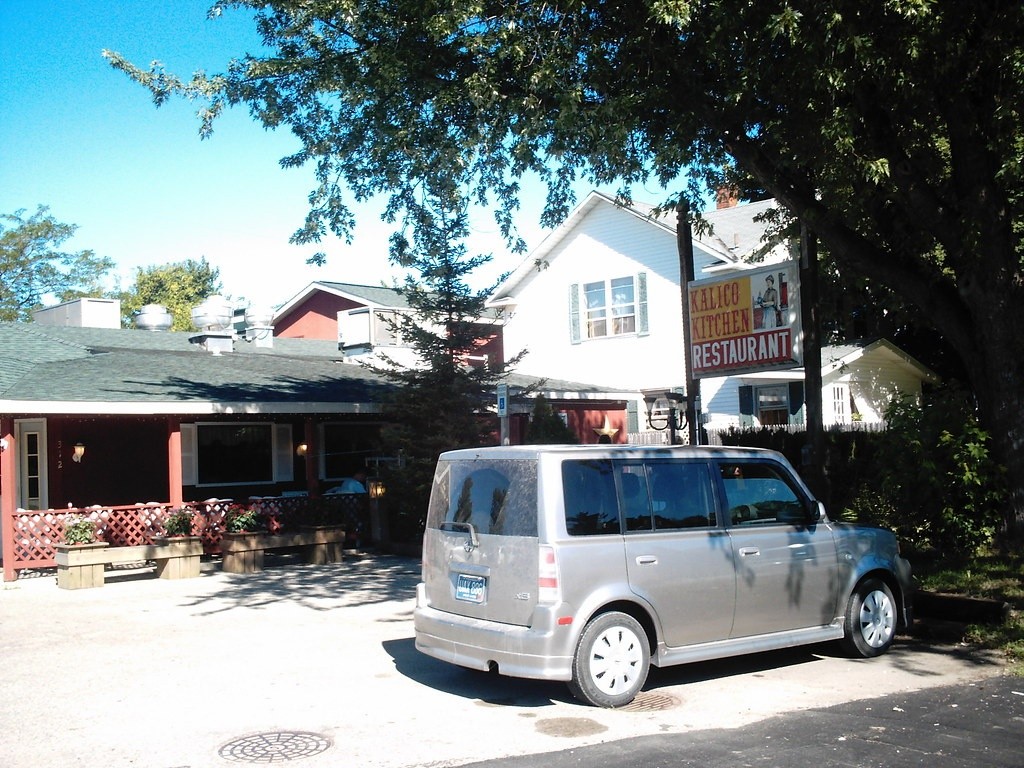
[72,442,85,463]
[295,439,309,455]
[365,477,387,500]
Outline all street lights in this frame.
[643,392,688,445]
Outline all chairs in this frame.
[653,473,704,522]
[602,473,642,525]
[571,469,601,534]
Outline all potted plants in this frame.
[51,517,110,587]
[219,505,266,572]
[292,493,346,564]
[150,509,204,577]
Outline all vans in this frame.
[413,445,914,709]
